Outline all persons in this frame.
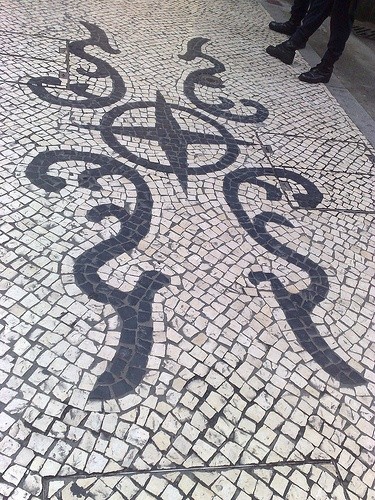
[268,1,315,49]
[265,1,358,84]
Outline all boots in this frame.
[298,60,334,83]
[269,15,301,36]
[266,40,296,65]
